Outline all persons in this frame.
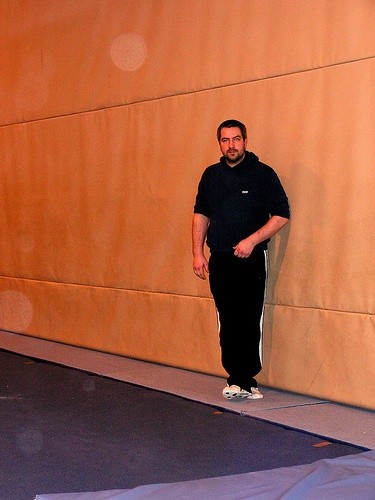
[192,119,291,398]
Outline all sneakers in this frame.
[222,385,264,400]
